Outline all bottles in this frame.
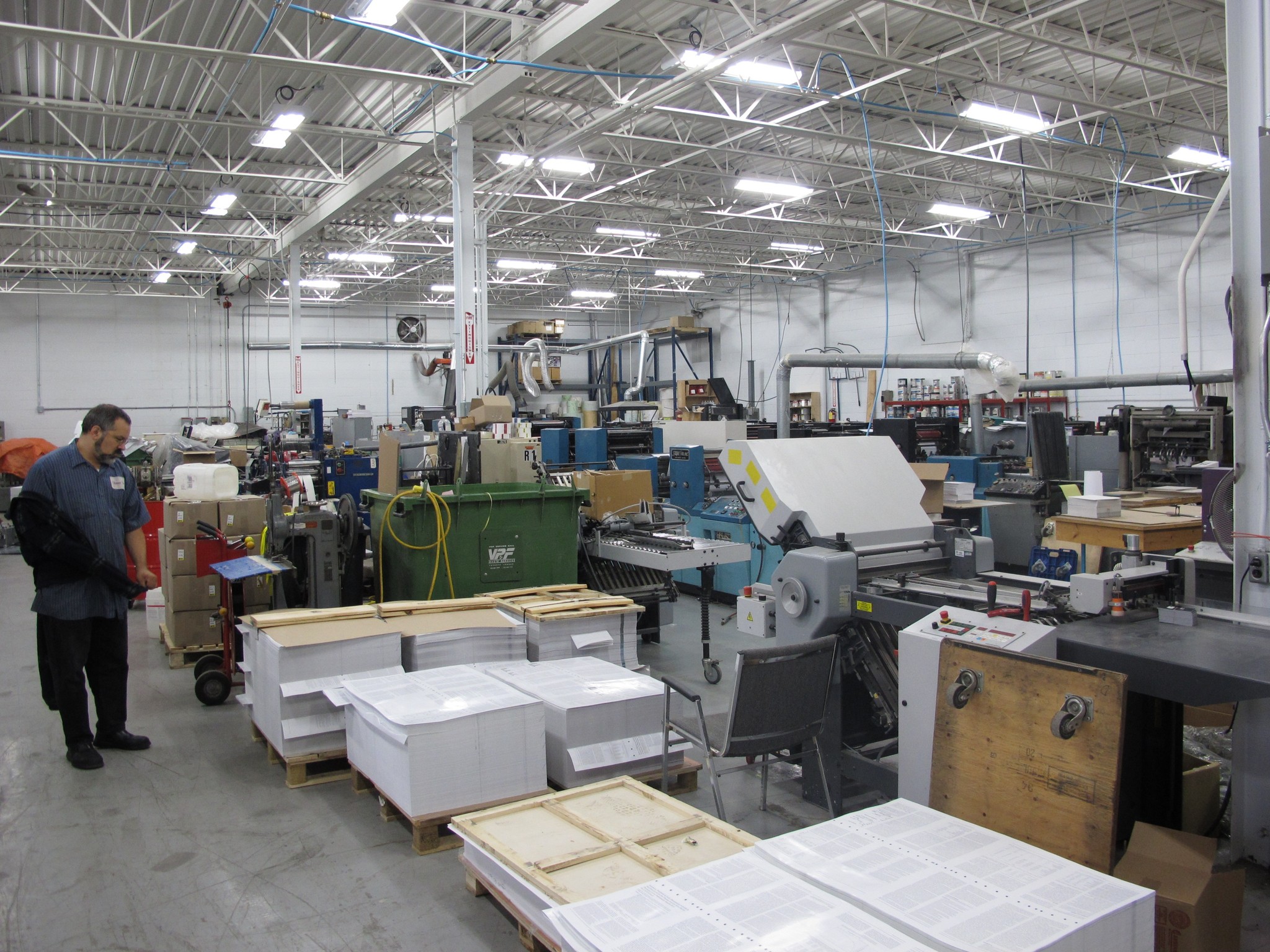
[415,419,424,432]
[701,400,715,414]
[438,416,453,432]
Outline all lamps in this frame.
[156,0,1230,292]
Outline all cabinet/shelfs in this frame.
[885,397,1068,422]
[790,392,821,422]
[676,380,718,408]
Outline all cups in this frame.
[675,410,682,421]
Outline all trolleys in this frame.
[194,517,247,705]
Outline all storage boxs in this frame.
[454,416,475,431]
[909,463,950,514]
[678,407,705,421]
[1113,820,1245,952]
[686,379,708,396]
[670,316,694,328]
[468,395,510,425]
[513,352,563,384]
[1182,751,1220,834]
[158,446,270,647]
[573,470,654,521]
[507,318,565,335]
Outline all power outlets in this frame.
[1248,552,1269,583]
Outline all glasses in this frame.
[102,429,128,445]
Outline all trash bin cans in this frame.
[117,449,153,497]
[124,501,163,608]
[359,474,592,604]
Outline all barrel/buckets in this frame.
[789,399,811,422]
[146,586,167,639]
[984,392,1012,420]
[1033,371,1064,397]
[887,377,968,422]
[173,462,239,503]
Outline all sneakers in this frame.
[67,744,104,769]
[93,730,150,749]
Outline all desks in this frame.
[1051,504,1202,572]
[943,499,1017,537]
[1103,491,1202,509]
[1175,541,1233,571]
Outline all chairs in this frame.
[662,634,838,823]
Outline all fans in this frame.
[396,315,425,343]
[23,166,63,208]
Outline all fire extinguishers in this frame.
[828,410,836,423]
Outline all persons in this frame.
[4,405,157,770]
